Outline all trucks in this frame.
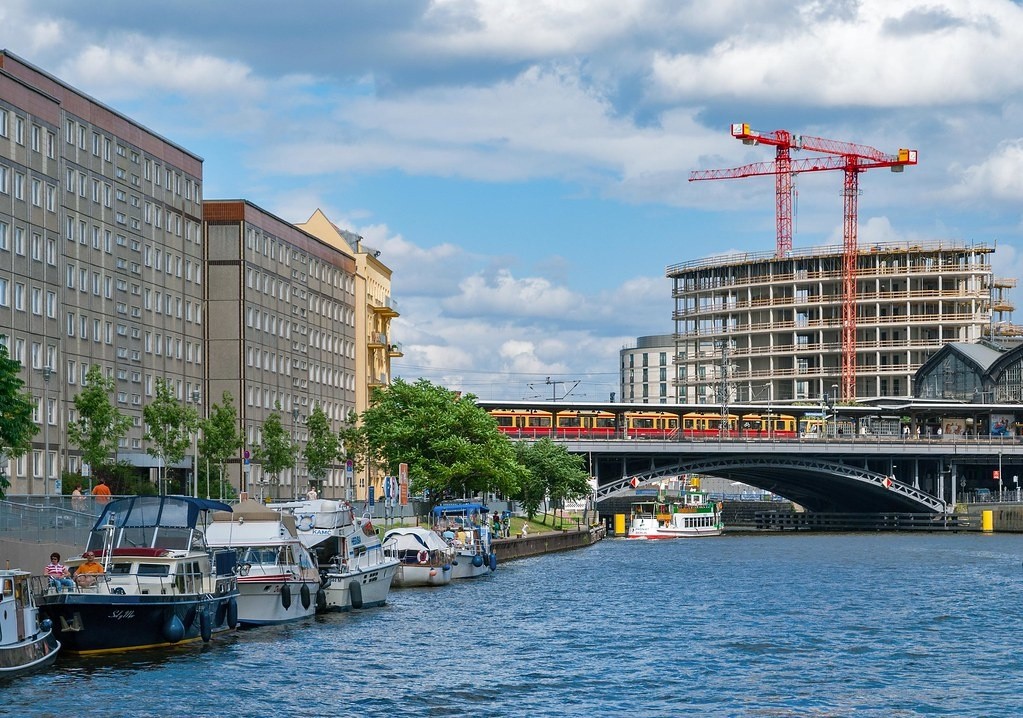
[967,487,991,500]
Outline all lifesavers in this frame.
[417,549,429,564]
[296,512,316,532]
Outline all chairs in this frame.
[43,567,108,595]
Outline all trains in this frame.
[488,407,799,439]
[799,419,828,437]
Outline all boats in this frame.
[30,494,241,660]
[0,568,62,682]
[626,490,726,538]
[380,525,458,587]
[203,492,335,626]
[430,504,497,579]
[276,499,402,614]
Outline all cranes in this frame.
[690,123,919,405]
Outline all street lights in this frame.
[291,407,300,503]
[42,365,51,495]
[765,381,771,438]
[997,448,1003,503]
[192,389,200,498]
[831,383,838,438]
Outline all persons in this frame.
[73,551,104,587]
[522,521,530,538]
[71,483,87,512]
[501,511,510,537]
[742,488,747,494]
[917,426,920,439]
[492,511,502,538]
[44,553,73,593]
[92,478,112,526]
[937,427,943,439]
[838,427,843,434]
[903,425,912,440]
[306,486,317,500]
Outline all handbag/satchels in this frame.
[494,523,499,530]
